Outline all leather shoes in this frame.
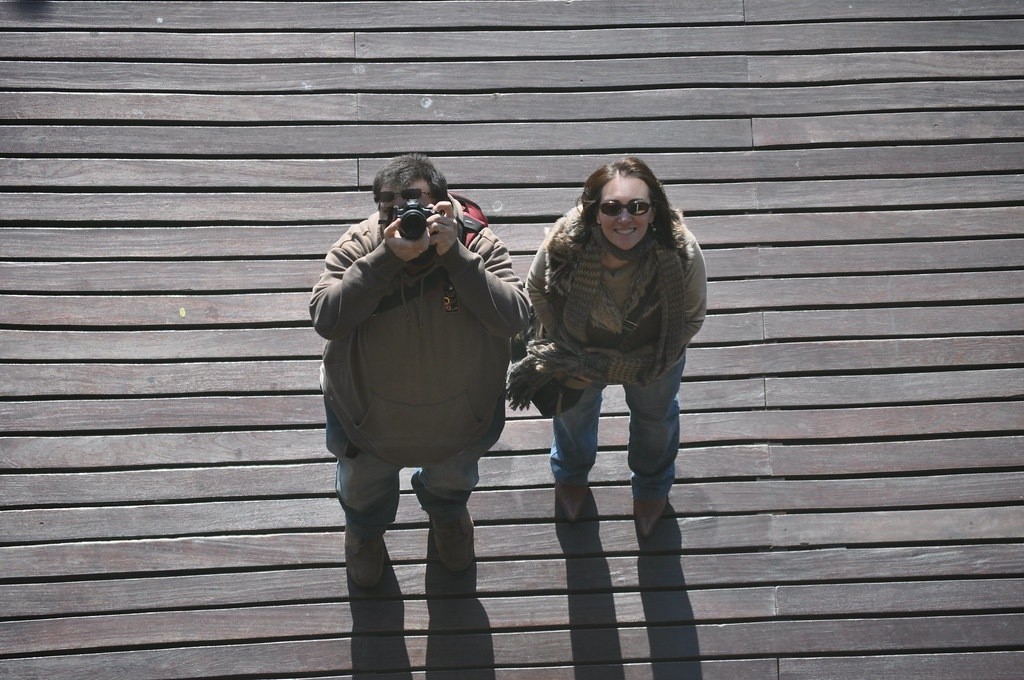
[634,497,664,538]
[556,478,588,522]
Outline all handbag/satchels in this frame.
[510,305,587,417]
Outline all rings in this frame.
[418,249,422,253]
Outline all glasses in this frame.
[595,198,655,216]
[374,188,434,203]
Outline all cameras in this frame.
[389,199,444,241]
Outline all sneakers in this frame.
[344,522,385,587]
[411,471,474,571]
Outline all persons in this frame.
[308,153,529,588]
[525,157,708,537]
